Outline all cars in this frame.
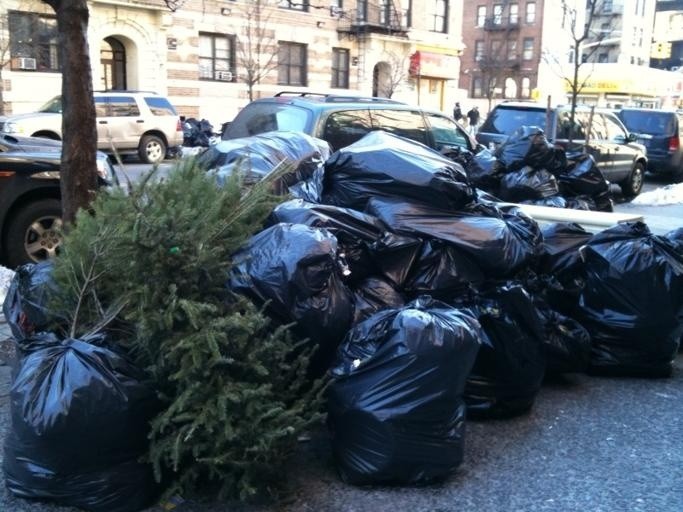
[621,108,682,179]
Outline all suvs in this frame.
[225,90,488,156]
[3,90,183,163]
[1,130,120,274]
[475,98,649,199]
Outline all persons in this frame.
[454,101,462,121]
[467,105,480,135]
